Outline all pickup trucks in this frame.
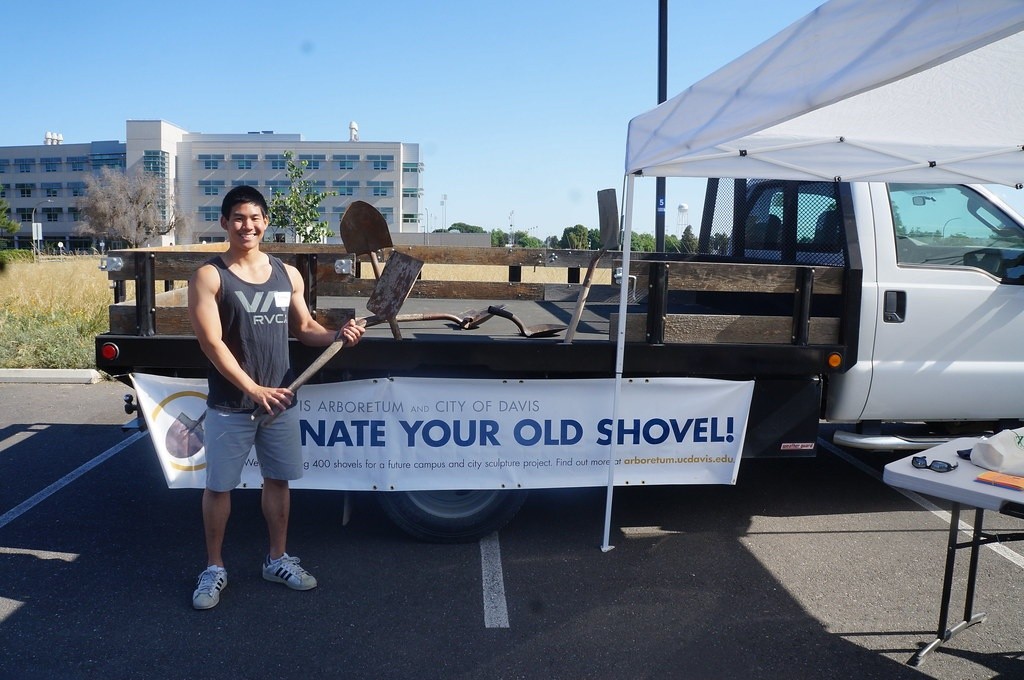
[96,163,1024,542]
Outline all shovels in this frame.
[339,198,404,341]
[356,303,508,329]
[249,248,426,422]
[487,305,569,337]
[562,188,622,343]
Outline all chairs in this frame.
[813,211,844,245]
[764,214,781,249]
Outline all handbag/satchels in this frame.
[970,427,1024,477]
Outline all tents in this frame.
[598,0,1024,554]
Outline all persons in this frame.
[189,184,370,611]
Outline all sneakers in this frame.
[263,553,317,590]
[193,565,227,609]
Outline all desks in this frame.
[882,437,1024,668]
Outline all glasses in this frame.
[912,456,959,472]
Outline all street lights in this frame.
[32,201,55,261]
